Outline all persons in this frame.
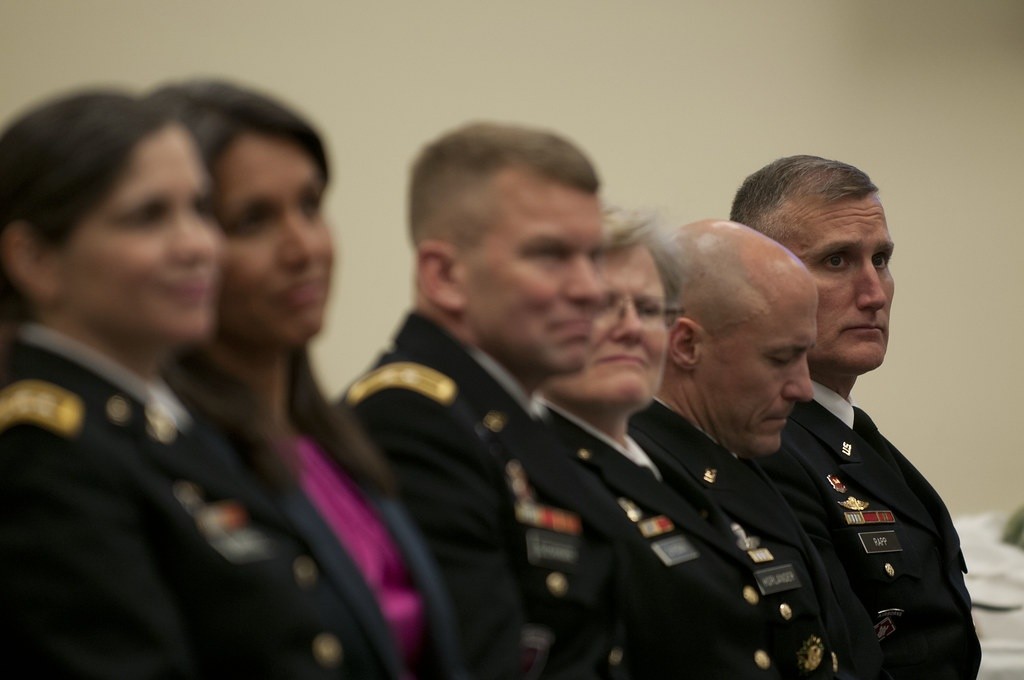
[625,218,859,680]
[0,88,390,680]
[527,207,782,680]
[339,121,712,680]
[144,83,467,680]
[728,155,981,680]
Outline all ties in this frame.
[852,407,906,484]
[641,464,657,481]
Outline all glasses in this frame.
[587,287,683,329]
